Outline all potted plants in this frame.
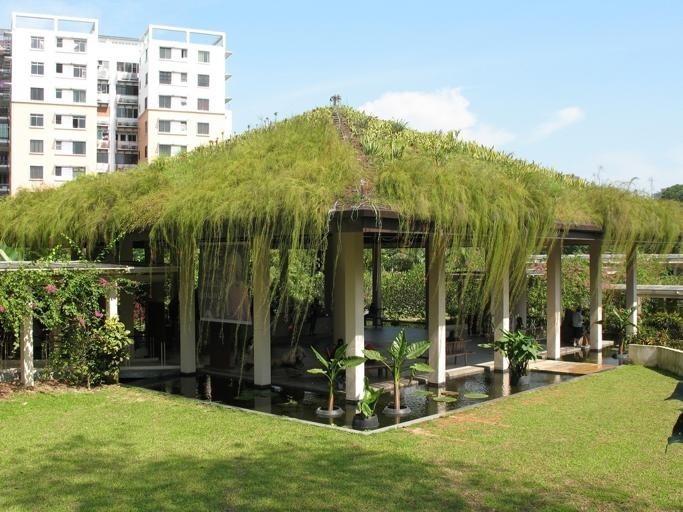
[477,327,544,387]
[304,331,436,431]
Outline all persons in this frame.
[572,305,585,348]
